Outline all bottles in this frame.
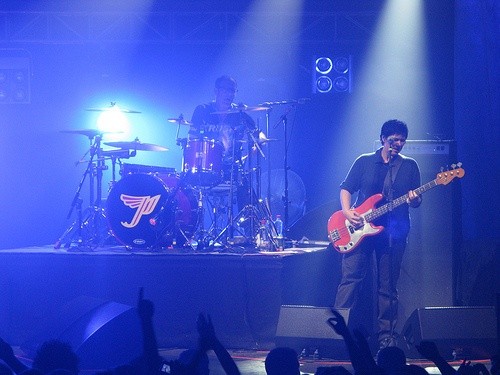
[259,215,283,253]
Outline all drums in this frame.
[121,161,178,189]
[192,162,241,195]
[106,173,200,251]
[182,138,223,186]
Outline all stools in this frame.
[194,183,237,246]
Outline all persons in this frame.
[336,120,424,351]
[0,288,500,375]
[190,75,266,246]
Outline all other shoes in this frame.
[376,339,395,358]
[183,235,199,246]
[208,240,222,247]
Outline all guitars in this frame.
[327,162,465,254]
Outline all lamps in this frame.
[0,46,34,105]
[313,53,353,94]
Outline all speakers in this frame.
[275,304,354,359]
[397,155,459,309]
[22,296,145,375]
[400,305,498,358]
[198,194,240,237]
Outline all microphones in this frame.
[273,112,288,129]
[387,136,394,152]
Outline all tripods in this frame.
[52,113,284,252]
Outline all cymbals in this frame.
[210,107,270,115]
[103,141,168,151]
[169,117,193,125]
[238,137,278,143]
[60,127,125,135]
[85,107,142,113]
[98,149,135,157]
